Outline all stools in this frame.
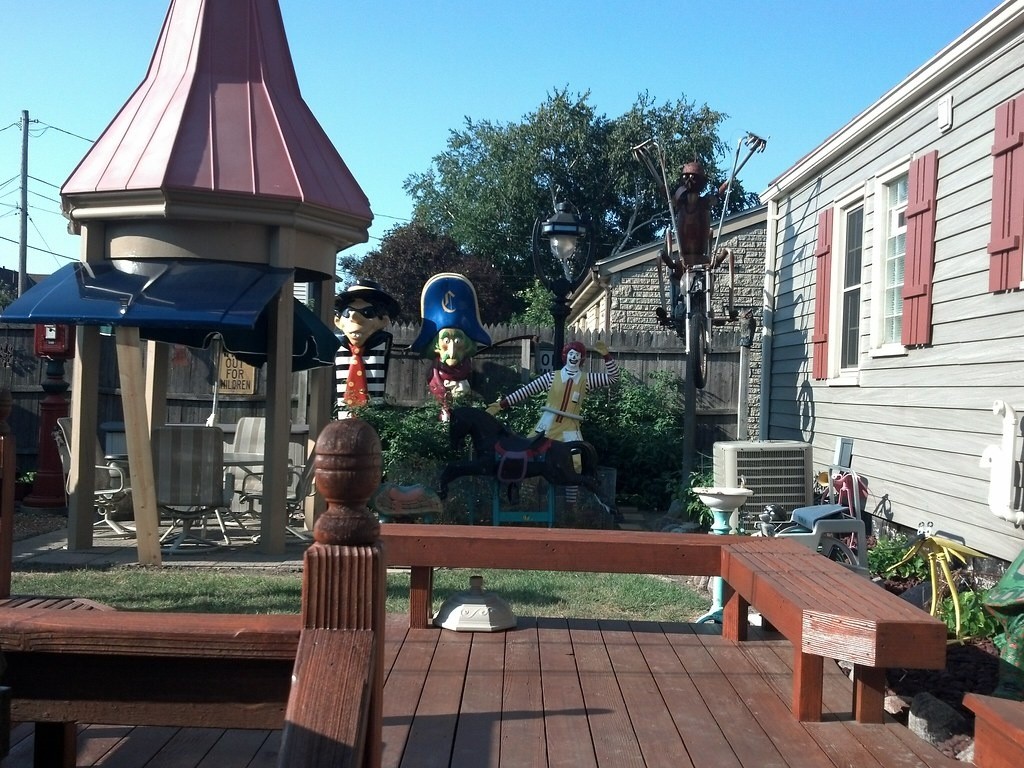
[777,467,869,579]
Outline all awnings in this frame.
[0,261,299,326]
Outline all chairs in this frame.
[147,423,230,554]
[228,441,317,542]
[54,415,135,540]
[218,416,266,528]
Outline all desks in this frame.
[107,454,268,540]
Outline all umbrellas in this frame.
[99,290,345,539]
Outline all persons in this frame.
[331,280,402,419]
[402,271,493,427]
[487,338,620,507]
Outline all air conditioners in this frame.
[713,439,814,533]
